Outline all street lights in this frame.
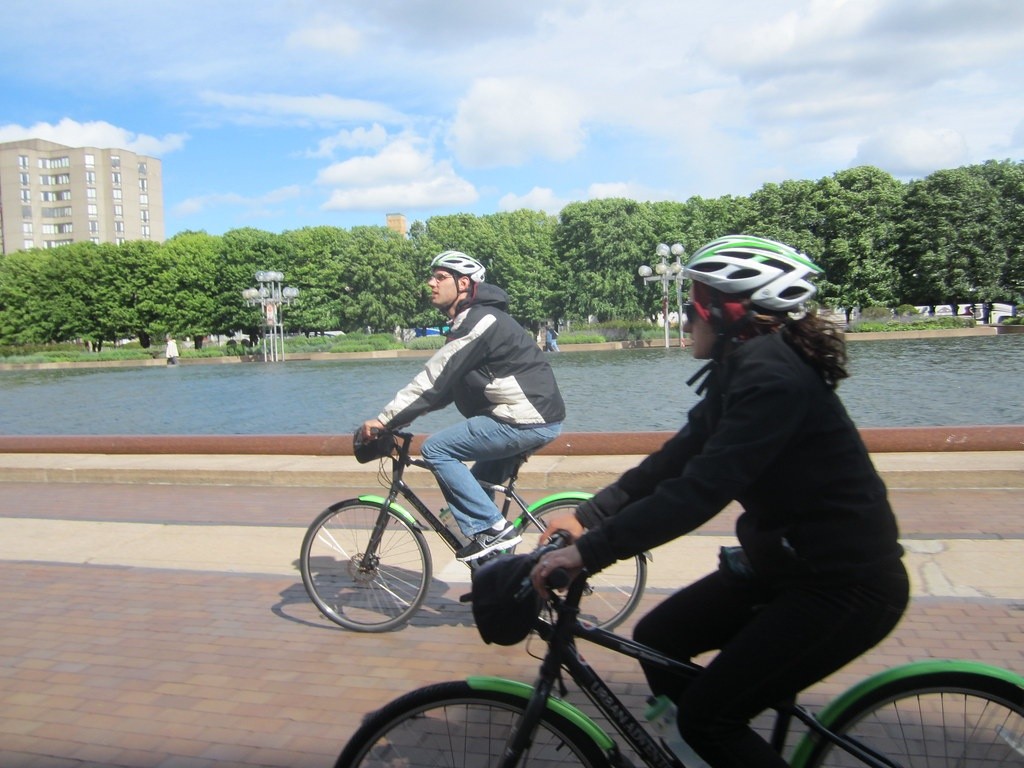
[243,269,300,362]
[638,241,693,349]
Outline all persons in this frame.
[164,333,182,368]
[532,236,908,768]
[353,249,568,604]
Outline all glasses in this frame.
[430,274,453,282]
[686,306,697,322]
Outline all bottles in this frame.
[439,507,471,549]
[642,693,710,767]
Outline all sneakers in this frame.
[455,521,522,561]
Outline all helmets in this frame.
[430,251,487,285]
[681,235,824,313]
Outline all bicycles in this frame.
[332,528,1023,768]
[301,426,651,635]
